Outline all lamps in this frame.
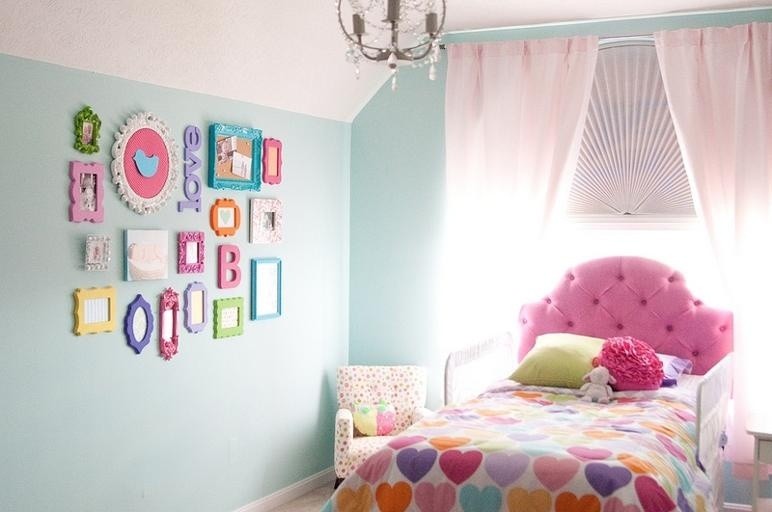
[336,0,448,92]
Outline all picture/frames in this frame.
[178,232,204,272]
[70,160,105,223]
[210,123,262,190]
[74,106,101,152]
[252,258,281,319]
[85,235,110,272]
[250,199,283,246]
[213,297,243,339]
[73,286,115,336]
[124,295,155,354]
[159,287,178,360]
[184,282,209,334]
[211,199,242,236]
[110,112,180,215]
[264,138,282,184]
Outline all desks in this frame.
[745,415,772,511]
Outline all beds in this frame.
[348,257,734,512]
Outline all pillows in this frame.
[506,332,692,389]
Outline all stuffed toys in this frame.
[580,366,615,405]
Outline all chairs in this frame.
[334,365,434,489]
